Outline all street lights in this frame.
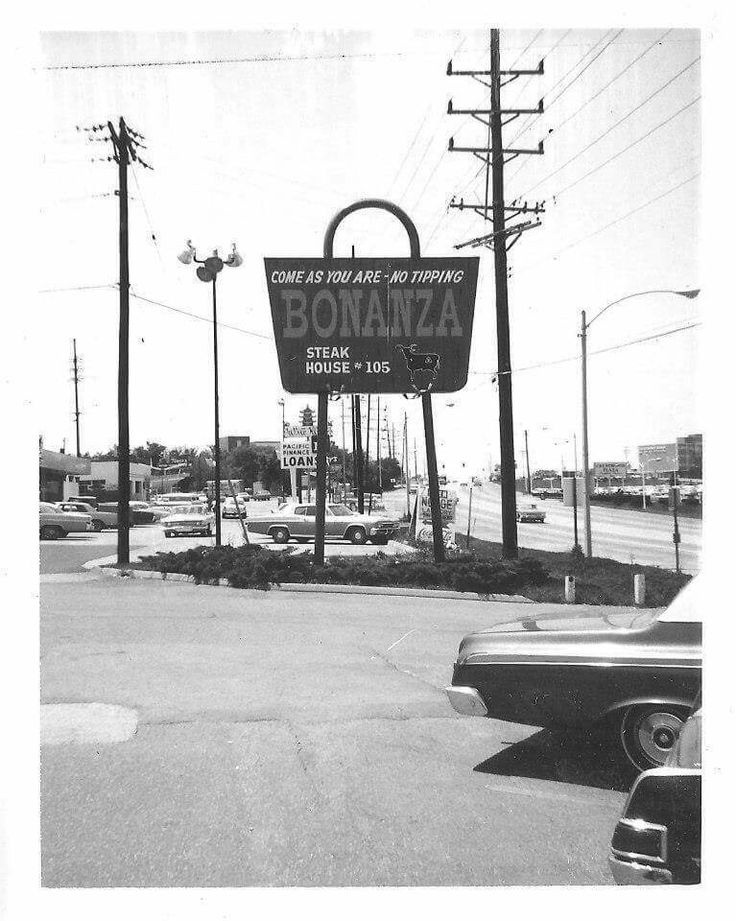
[277,399,285,497]
[178,243,245,544]
[629,458,662,509]
[580,282,700,555]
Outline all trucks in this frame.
[202,480,245,498]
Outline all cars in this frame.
[159,505,216,538]
[530,477,563,500]
[39,502,93,540]
[236,492,249,501]
[152,491,226,509]
[445,562,701,886]
[257,490,272,500]
[515,502,545,523]
[243,503,401,545]
[68,496,169,526]
[54,502,119,531]
[223,496,247,519]
[394,475,481,493]
[276,490,382,512]
